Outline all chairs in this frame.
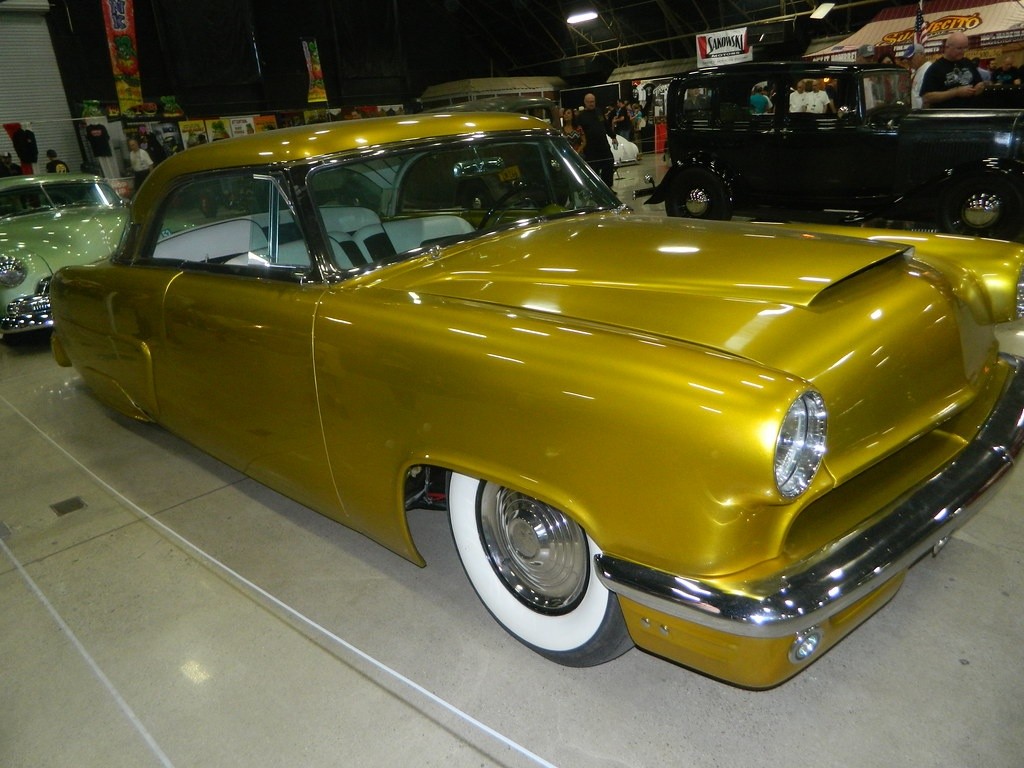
[157,204,479,281]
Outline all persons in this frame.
[130,141,154,190]
[683,88,711,111]
[920,32,984,109]
[141,139,148,150]
[751,55,916,115]
[557,94,642,195]
[899,32,1024,109]
[0,152,23,178]
[46,150,70,173]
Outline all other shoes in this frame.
[609,187,617,195]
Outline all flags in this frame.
[914,3,928,46]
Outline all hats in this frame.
[0,152,11,158]
[902,43,924,59]
[579,106,584,111]
[972,57,980,67]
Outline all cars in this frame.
[415,94,563,134]
[0,171,197,340]
[46,109,1024,694]
[605,133,641,166]
[628,56,1024,244]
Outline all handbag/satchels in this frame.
[638,117,646,127]
